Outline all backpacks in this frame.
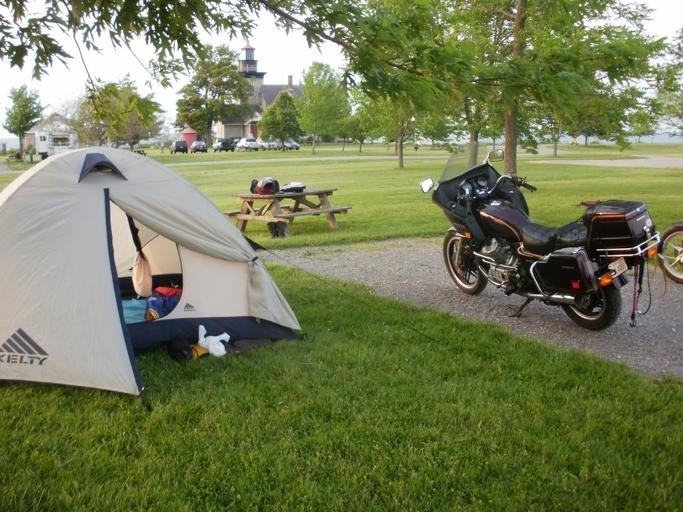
[144,292,181,321]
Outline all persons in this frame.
[170,145,173,154]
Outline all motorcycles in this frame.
[418,148,663,331]
[656,221,682,283]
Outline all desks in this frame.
[234,187,339,232]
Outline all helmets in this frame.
[256,177,279,195]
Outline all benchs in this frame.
[223,204,353,239]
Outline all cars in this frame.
[235,135,300,151]
[190,140,208,154]
[211,137,236,152]
[170,140,187,154]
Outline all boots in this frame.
[267,221,285,239]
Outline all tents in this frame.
[0,145,310,412]
